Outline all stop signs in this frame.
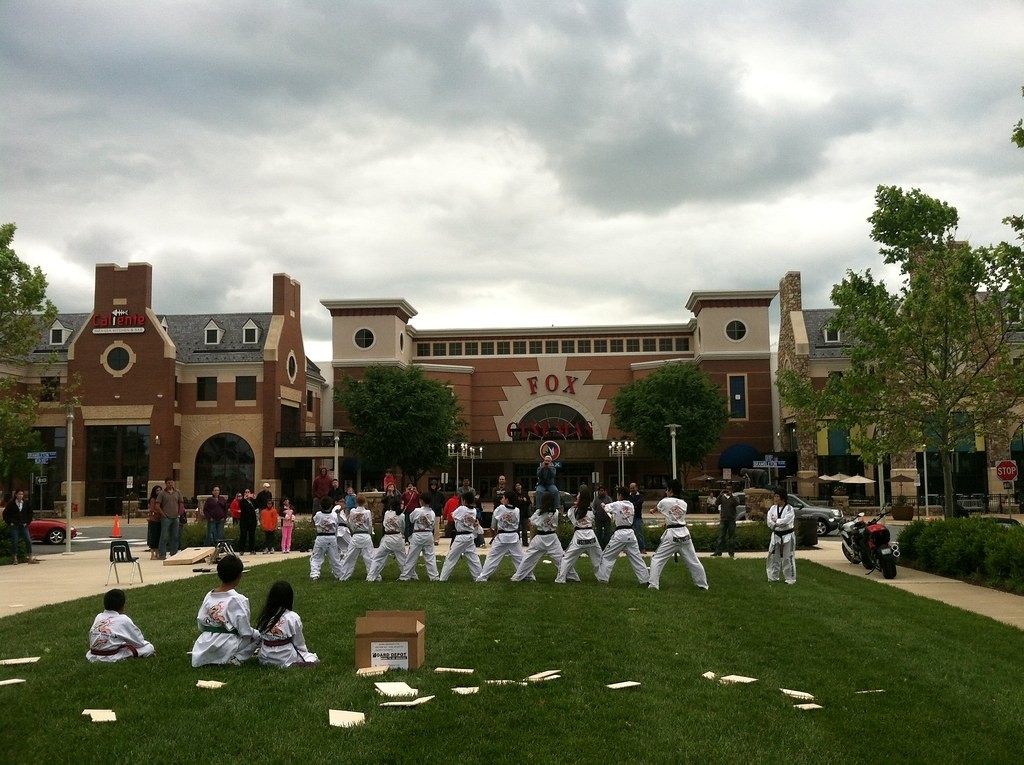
[995,460,1017,482]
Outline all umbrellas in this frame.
[783,473,914,496]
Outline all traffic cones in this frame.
[109,514,123,539]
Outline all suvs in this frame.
[718,491,844,537]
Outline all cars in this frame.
[27,517,77,545]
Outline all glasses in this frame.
[238,496,242,497]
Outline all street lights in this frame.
[447,443,468,492]
[608,442,634,501]
[664,423,682,481]
[463,446,483,489]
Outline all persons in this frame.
[277,495,295,553]
[474,519,485,548]
[331,494,351,560]
[396,493,440,581]
[381,482,402,538]
[311,466,357,523]
[230,486,259,556]
[428,479,446,546]
[86,553,319,669]
[384,468,396,491]
[339,494,382,581]
[3,490,40,565]
[155,476,184,559]
[203,485,228,547]
[255,482,272,520]
[365,496,420,581]
[475,489,537,582]
[260,498,279,554]
[440,491,483,581]
[473,489,485,548]
[456,476,476,506]
[147,485,162,560]
[402,481,420,546]
[309,497,342,580]
[442,487,460,537]
[492,456,796,589]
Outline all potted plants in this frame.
[681,491,701,514]
[892,496,914,520]
[833,486,847,495]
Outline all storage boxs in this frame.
[163,547,215,564]
[355,610,425,667]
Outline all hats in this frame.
[263,483,270,488]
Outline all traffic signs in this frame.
[35,476,48,485]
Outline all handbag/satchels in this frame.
[180,512,188,524]
[148,511,160,522]
[255,509,260,520]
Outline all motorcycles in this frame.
[836,511,900,580]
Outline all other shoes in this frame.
[729,552,734,557]
[250,550,256,554]
[262,548,268,554]
[28,558,38,564]
[14,557,18,563]
[286,549,289,552]
[282,550,286,554]
[270,548,275,554]
[240,550,244,555]
[710,551,722,557]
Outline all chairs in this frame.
[105,541,143,585]
[209,532,236,565]
